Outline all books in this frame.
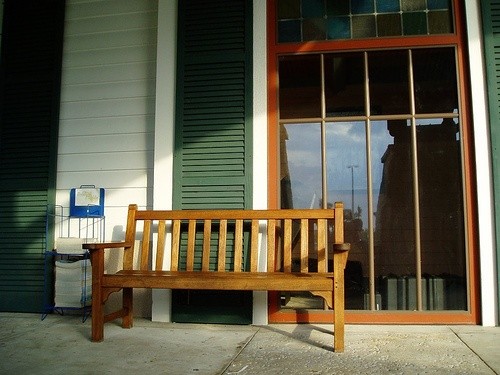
[54,259,92,308]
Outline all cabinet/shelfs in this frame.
[42,183,106,324]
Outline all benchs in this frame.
[82,201,350,353]
